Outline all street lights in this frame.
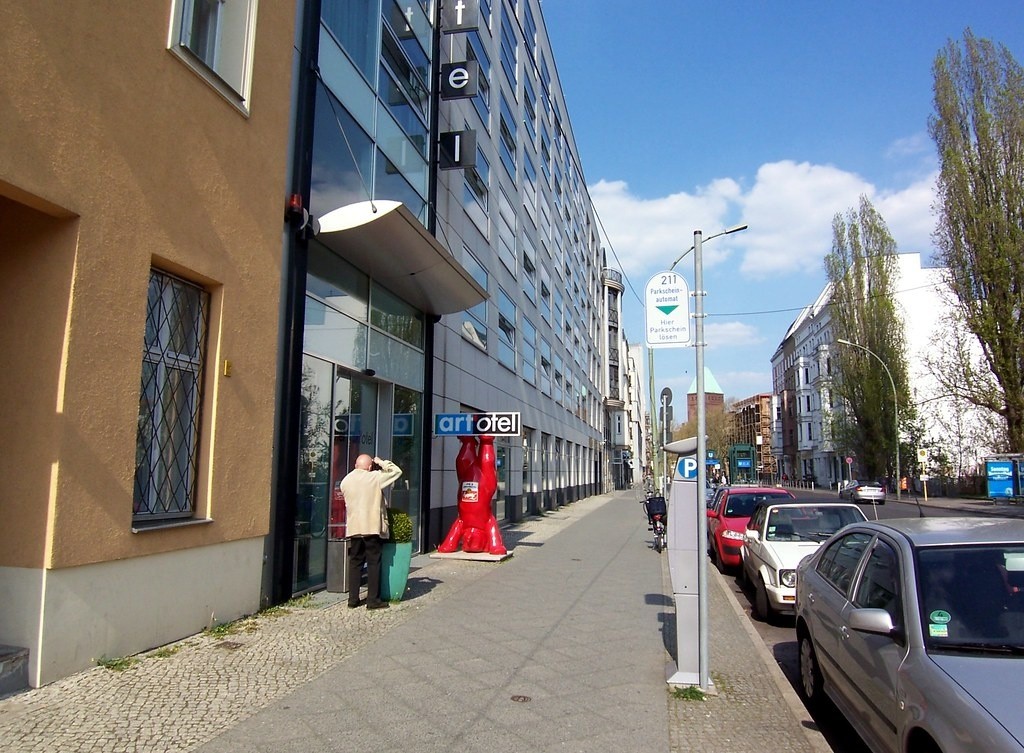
[834,336,903,502]
[665,223,750,696]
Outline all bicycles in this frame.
[639,481,667,556]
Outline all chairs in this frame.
[771,514,793,533]
[729,497,754,515]
[818,515,840,531]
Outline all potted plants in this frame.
[379,507,414,601]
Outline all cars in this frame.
[740,496,881,622]
[795,516,1023,753]
[706,480,753,515]
[839,479,887,505]
[708,488,810,574]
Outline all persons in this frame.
[340,454,402,610]
[437,436,508,555]
[712,474,726,493]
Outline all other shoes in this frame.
[348,600,364,608]
[367,601,390,611]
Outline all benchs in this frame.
[793,519,817,530]
